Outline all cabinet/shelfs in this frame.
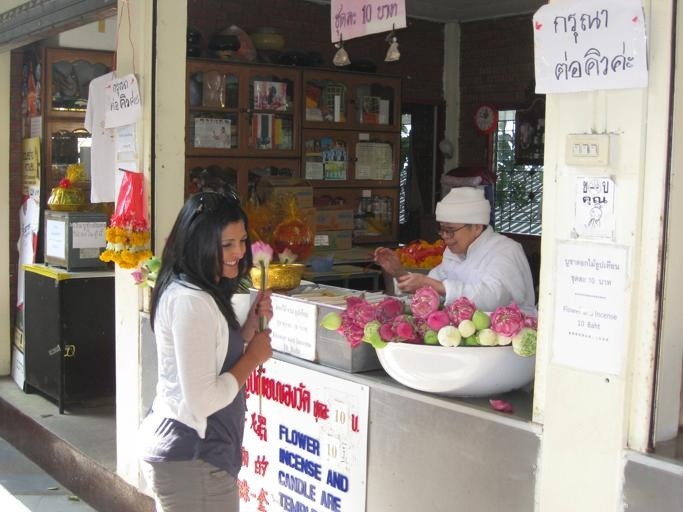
[46,47,117,207]
[23,263,115,414]
[185,59,401,247]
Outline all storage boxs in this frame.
[43,211,110,272]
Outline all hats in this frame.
[435,187,491,225]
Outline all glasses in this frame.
[436,225,468,238]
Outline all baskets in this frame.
[49,203,81,211]
[249,263,305,291]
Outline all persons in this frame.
[372,184,538,318]
[131,189,275,511]
